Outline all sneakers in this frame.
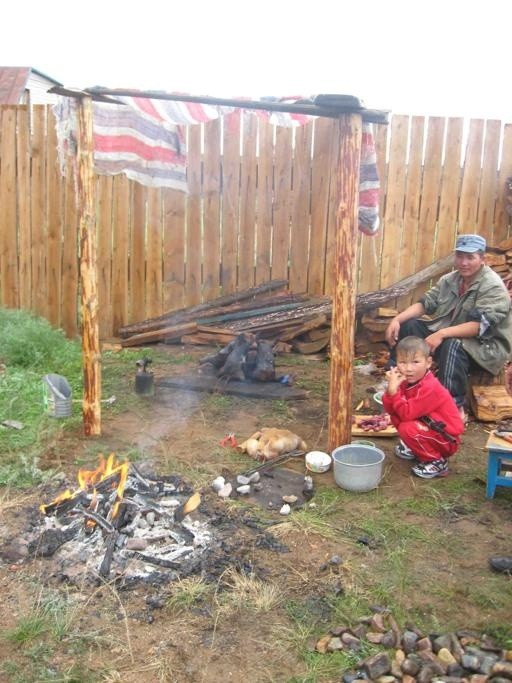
[412,458,449,479]
[396,444,416,461]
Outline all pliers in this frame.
[494,430,512,443]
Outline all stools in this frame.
[484,429,512,499]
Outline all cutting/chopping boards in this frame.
[350,414,399,437]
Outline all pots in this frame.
[332,443,386,492]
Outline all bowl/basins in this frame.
[305,450,333,474]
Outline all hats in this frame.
[455,235,486,253]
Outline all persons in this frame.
[382,336,464,478]
[385,234,512,424]
